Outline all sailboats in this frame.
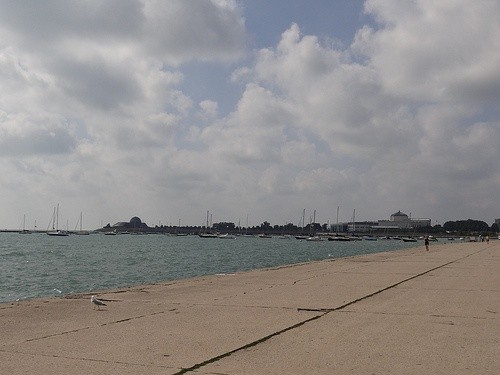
[18,214,32,235]
[46,204,69,236]
[91,207,482,243]
[75,212,90,236]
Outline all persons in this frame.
[425,235,429,252]
[479,234,490,245]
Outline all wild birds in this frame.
[91,296,107,310]
